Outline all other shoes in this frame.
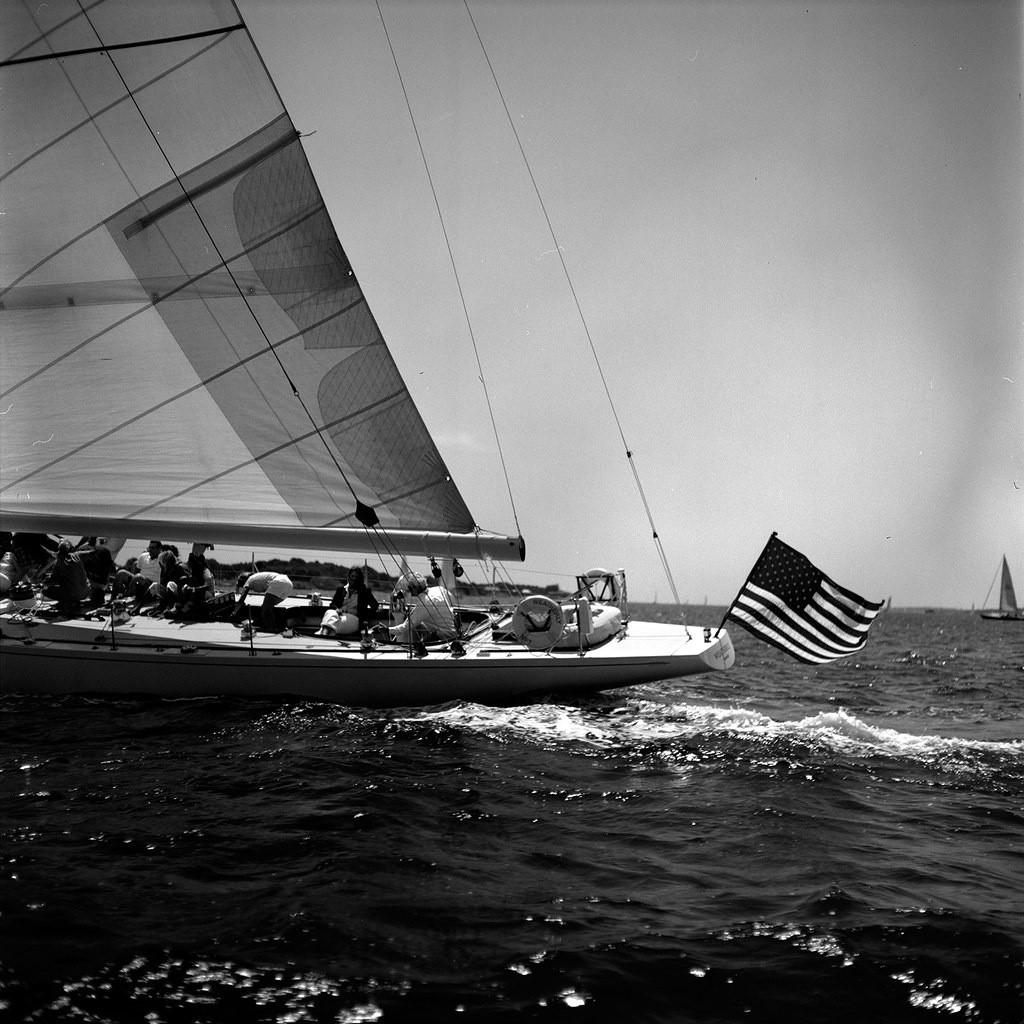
[130,608,139,615]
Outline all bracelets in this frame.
[232,612,235,616]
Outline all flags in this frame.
[725,536,885,666]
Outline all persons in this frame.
[11,532,58,570]
[106,541,162,606]
[83,547,117,609]
[377,572,459,640]
[229,571,293,626]
[129,545,215,619]
[42,538,91,620]
[313,567,380,636]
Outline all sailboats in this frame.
[0,0,735,700]
[980,555,1024,621]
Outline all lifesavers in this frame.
[579,569,621,608]
[511,595,565,649]
